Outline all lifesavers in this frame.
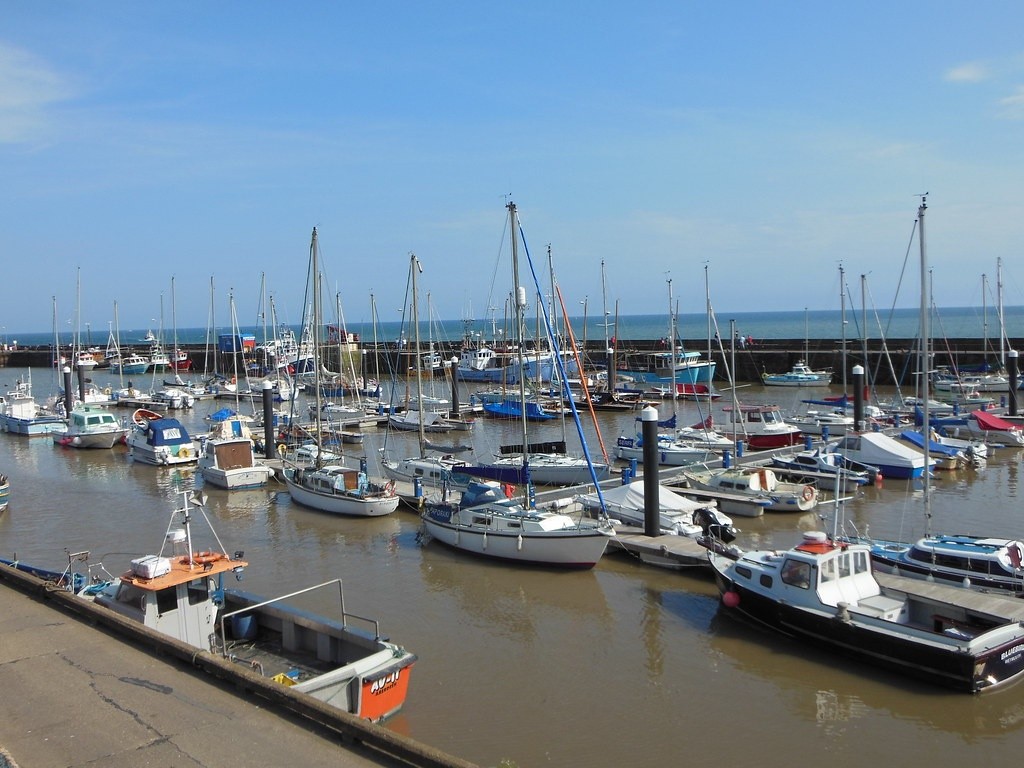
[277,444,287,455]
[184,550,220,564]
[802,486,815,501]
[385,482,396,497]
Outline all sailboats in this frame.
[365,290,497,510]
[48,183,1023,534]
[817,186,1022,608]
[282,227,403,517]
[380,251,519,489]
[49,268,131,450]
[422,198,616,573]
[697,262,1023,696]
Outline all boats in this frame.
[201,407,271,489]
[7,491,418,726]
[0,364,68,434]
[124,408,205,468]
